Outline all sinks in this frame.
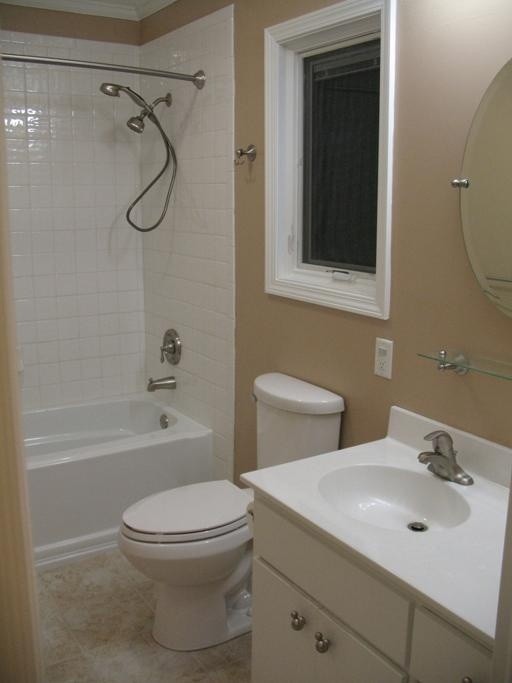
[318,466,470,538]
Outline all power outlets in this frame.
[374,336,394,380]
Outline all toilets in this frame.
[119,371,346,651]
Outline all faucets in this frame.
[417,429,476,487]
[146,376,176,391]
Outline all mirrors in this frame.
[458,57,511,316]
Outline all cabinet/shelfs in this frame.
[250,492,496,683]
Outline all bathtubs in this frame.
[20,400,213,570]
